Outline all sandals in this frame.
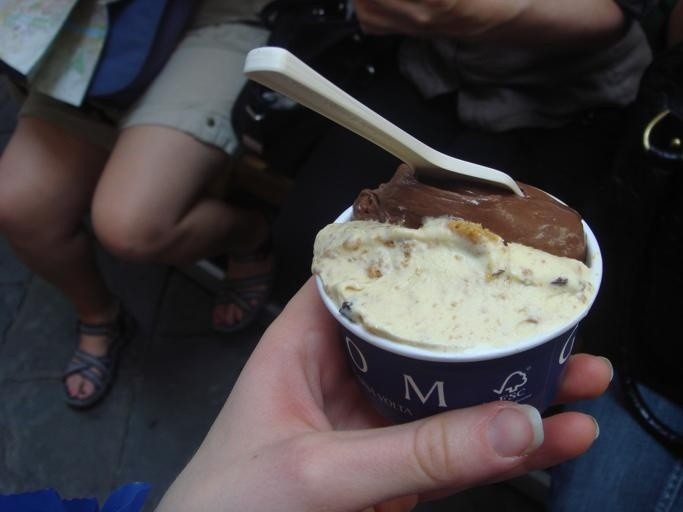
[62,298,134,406]
[216,240,278,333]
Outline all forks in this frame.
[241,45,524,202]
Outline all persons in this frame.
[231,1,683,305]
[152,272,614,512]
[1,1,292,408]
